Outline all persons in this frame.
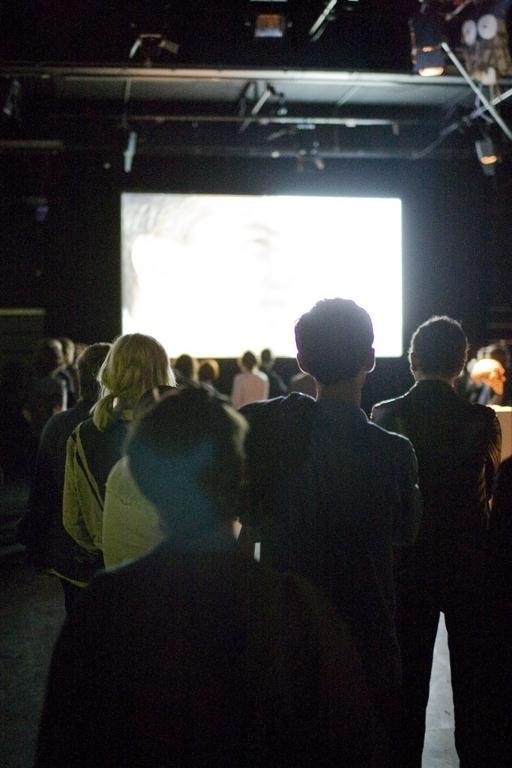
[233,296,424,767]
[33,384,397,766]
[369,314,510,768]
